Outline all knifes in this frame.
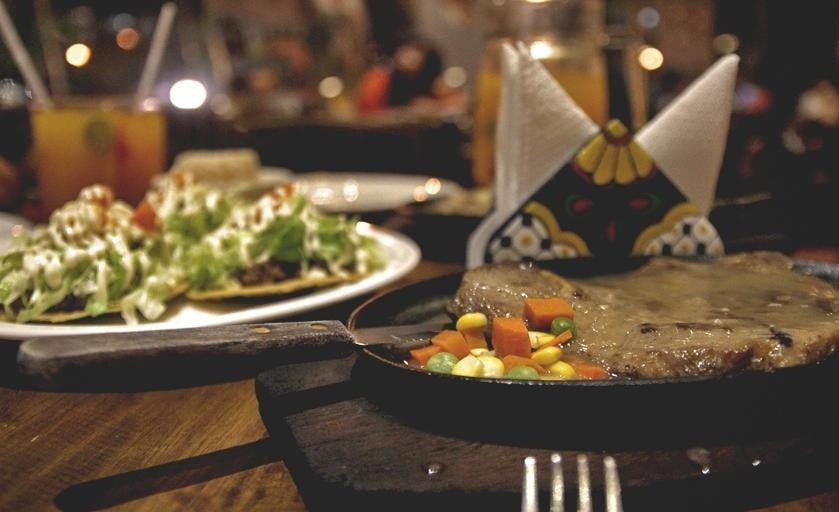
[19,319,455,386]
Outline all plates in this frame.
[0,221,424,342]
[347,253,838,428]
[292,166,459,218]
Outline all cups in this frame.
[469,35,611,190]
[23,94,169,218]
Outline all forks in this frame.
[520,454,624,512]
